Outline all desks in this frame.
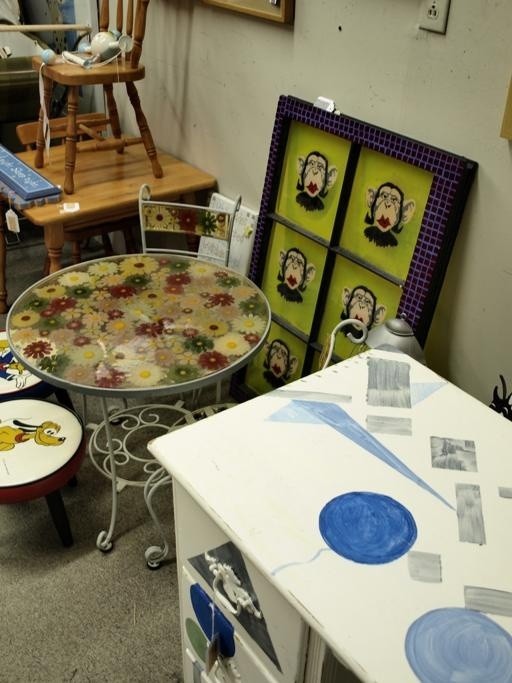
[2,134,218,275]
[148,342,511,683]
[5,250,271,569]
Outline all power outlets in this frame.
[416,0,450,35]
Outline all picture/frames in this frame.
[227,94,478,404]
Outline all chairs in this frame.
[140,184,241,411]
[16,111,142,264]
[31,0,162,194]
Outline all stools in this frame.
[0,328,84,550]
